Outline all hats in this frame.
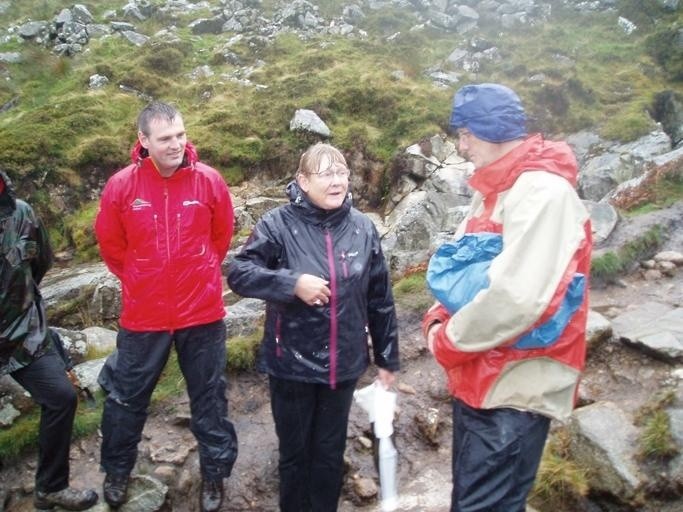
[449,83,528,143]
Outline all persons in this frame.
[0,170,98,512]
[98,102,240,510]
[224,142,400,512]
[424,83,592,512]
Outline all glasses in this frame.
[311,169,350,178]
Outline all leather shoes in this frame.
[201,479,222,512]
[33,487,98,510]
[103,473,129,506]
